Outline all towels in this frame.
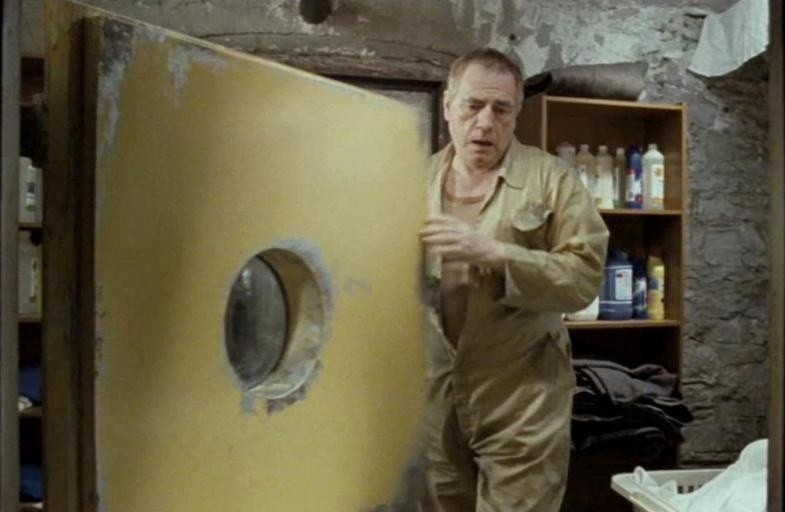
[684,0,769,81]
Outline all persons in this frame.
[418,46,612,511]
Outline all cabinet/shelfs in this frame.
[517,95,690,511]
[16,51,51,511]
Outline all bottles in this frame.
[555,140,666,323]
[16,156,44,315]
